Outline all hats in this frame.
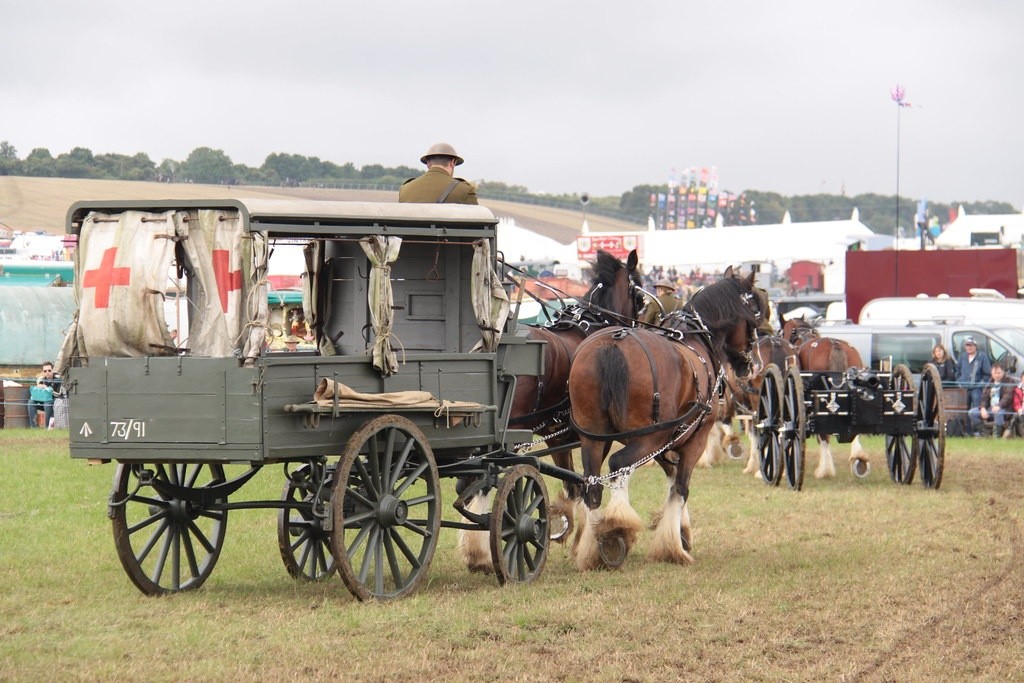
[964,338,977,345]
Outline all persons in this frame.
[289,310,299,335]
[399,144,479,206]
[170,330,177,341]
[929,337,1024,439]
[512,256,539,278]
[28,362,65,428]
[640,273,709,328]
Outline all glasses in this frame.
[43,370,51,372]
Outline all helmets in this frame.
[653,279,675,292]
[420,143,464,166]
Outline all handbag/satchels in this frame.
[30,386,53,402]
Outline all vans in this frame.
[812,320,1024,395]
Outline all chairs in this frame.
[984,388,1022,437]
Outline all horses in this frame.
[692,311,874,485]
[454,246,773,574]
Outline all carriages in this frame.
[746,305,948,492]
[63,196,767,608]
